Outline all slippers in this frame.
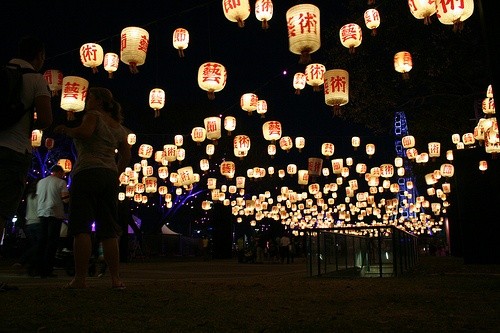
[113,281,126,289]
[64,282,87,290]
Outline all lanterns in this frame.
[32,0,500,237]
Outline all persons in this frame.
[0,39,301,292]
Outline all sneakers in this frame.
[0,280,18,294]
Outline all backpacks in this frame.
[0,62,44,131]
